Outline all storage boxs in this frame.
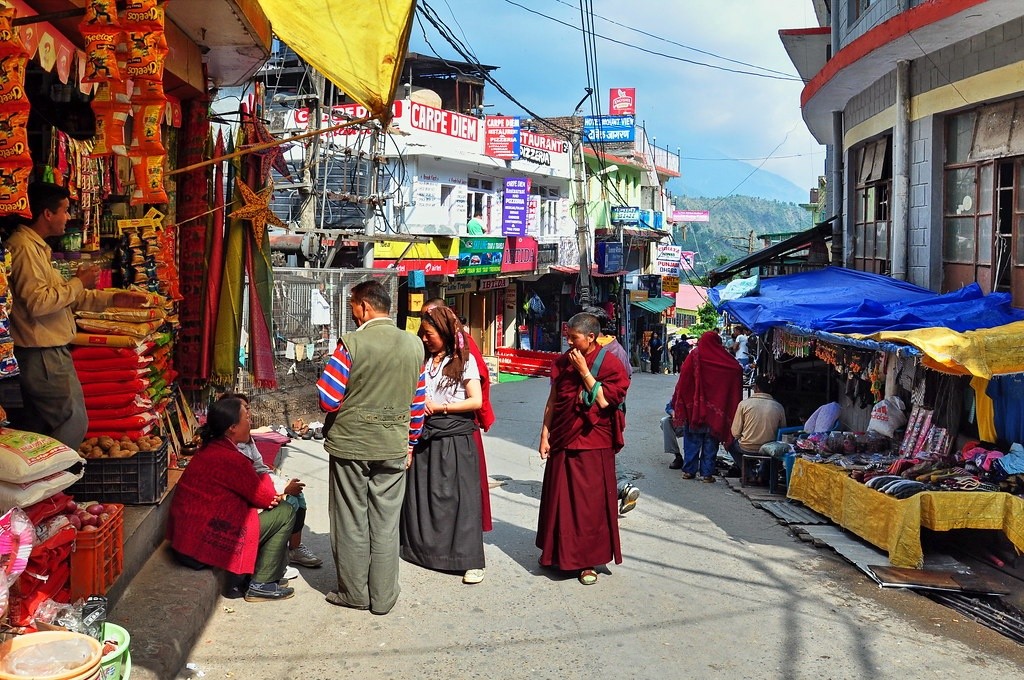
[65,504,125,595]
[62,436,169,507]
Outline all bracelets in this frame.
[442,404,447,414]
[582,372,590,380]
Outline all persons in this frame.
[417,298,495,532]
[1,182,148,452]
[315,280,425,615]
[399,306,486,584]
[535,312,630,584]
[648,331,690,376]
[660,325,814,483]
[467,211,487,235]
[165,394,320,602]
[604,295,617,318]
[595,315,639,515]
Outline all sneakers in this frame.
[244,583,294,602]
[289,543,320,568]
[277,579,288,587]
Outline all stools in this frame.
[742,455,772,488]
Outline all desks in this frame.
[786,456,1024,569]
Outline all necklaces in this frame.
[429,356,443,379]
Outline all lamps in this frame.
[526,118,531,125]
[404,82,411,91]
[477,105,485,113]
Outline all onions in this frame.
[64,500,117,531]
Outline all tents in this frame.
[706,265,1024,380]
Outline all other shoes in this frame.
[683,473,695,479]
[579,566,597,585]
[669,457,683,468]
[327,590,370,609]
[620,483,639,513]
[538,555,543,565]
[464,569,484,583]
[704,476,715,483]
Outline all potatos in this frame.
[78,436,163,458]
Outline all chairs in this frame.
[776,420,839,490]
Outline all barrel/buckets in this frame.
[0,621,132,680]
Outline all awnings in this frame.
[122,0,415,226]
[270,234,364,249]
[572,265,630,277]
[632,294,675,313]
[705,216,838,288]
[550,266,579,274]
